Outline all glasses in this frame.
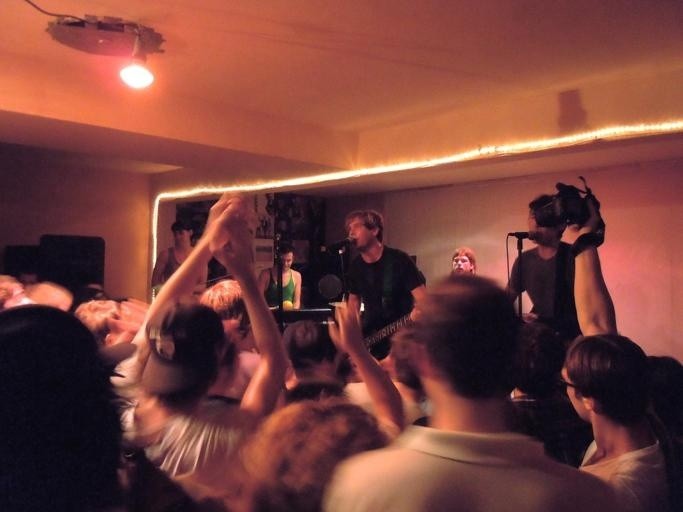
[557,377,583,397]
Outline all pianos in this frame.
[269,309,337,325]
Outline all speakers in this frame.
[310,241,351,305]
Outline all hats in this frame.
[138,299,227,401]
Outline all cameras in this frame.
[529,183,591,227]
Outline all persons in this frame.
[1,193,683,512]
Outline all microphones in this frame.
[320,237,354,253]
[509,231,536,240]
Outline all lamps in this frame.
[119,35,154,90]
